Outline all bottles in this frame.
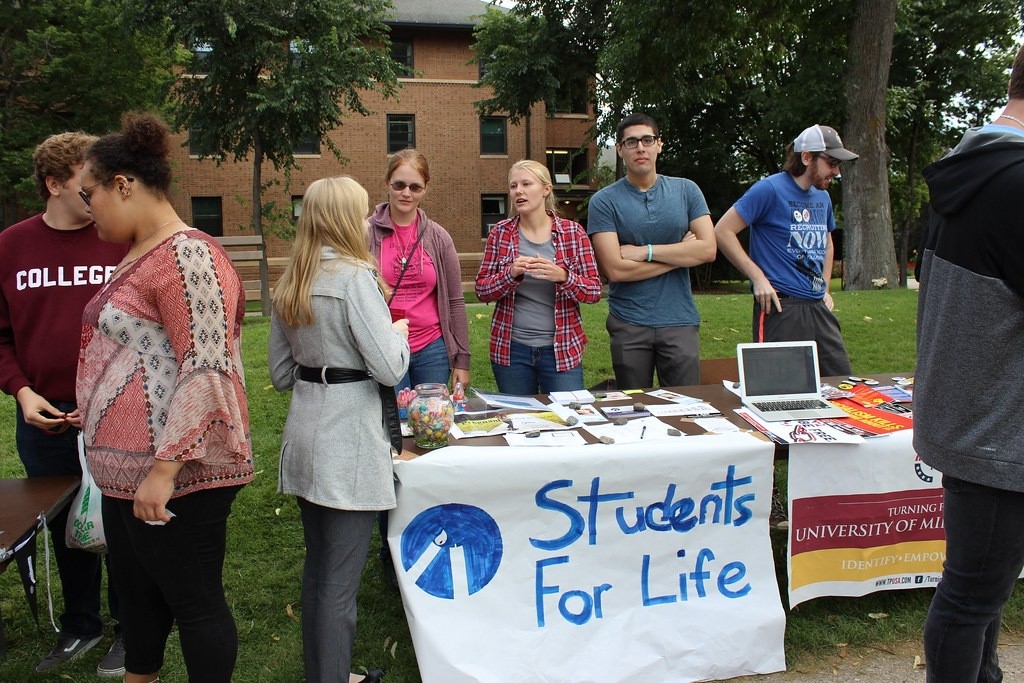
[407,383,455,449]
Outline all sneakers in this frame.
[97,635,126,678]
[769,487,788,530]
[36,633,104,673]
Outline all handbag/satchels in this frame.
[65,430,108,552]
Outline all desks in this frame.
[0,474,81,576]
[386,372,946,683]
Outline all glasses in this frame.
[619,135,660,149]
[389,179,426,193]
[77,176,134,206]
[814,153,843,169]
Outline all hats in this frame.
[793,124,860,161]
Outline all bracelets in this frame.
[647,244,652,261]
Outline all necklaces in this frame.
[131,220,182,249]
[394,220,415,263]
[1000,115,1024,127]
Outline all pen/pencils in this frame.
[687,413,724,418]
[641,426,646,439]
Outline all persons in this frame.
[0,132,132,676]
[364,149,471,399]
[716,124,860,377]
[587,113,716,391]
[576,409,594,415]
[912,46,1024,683]
[475,160,602,395]
[268,177,411,683]
[76,114,255,683]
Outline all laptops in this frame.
[735,341,848,422]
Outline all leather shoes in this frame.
[358,670,383,683]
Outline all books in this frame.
[601,405,650,418]
